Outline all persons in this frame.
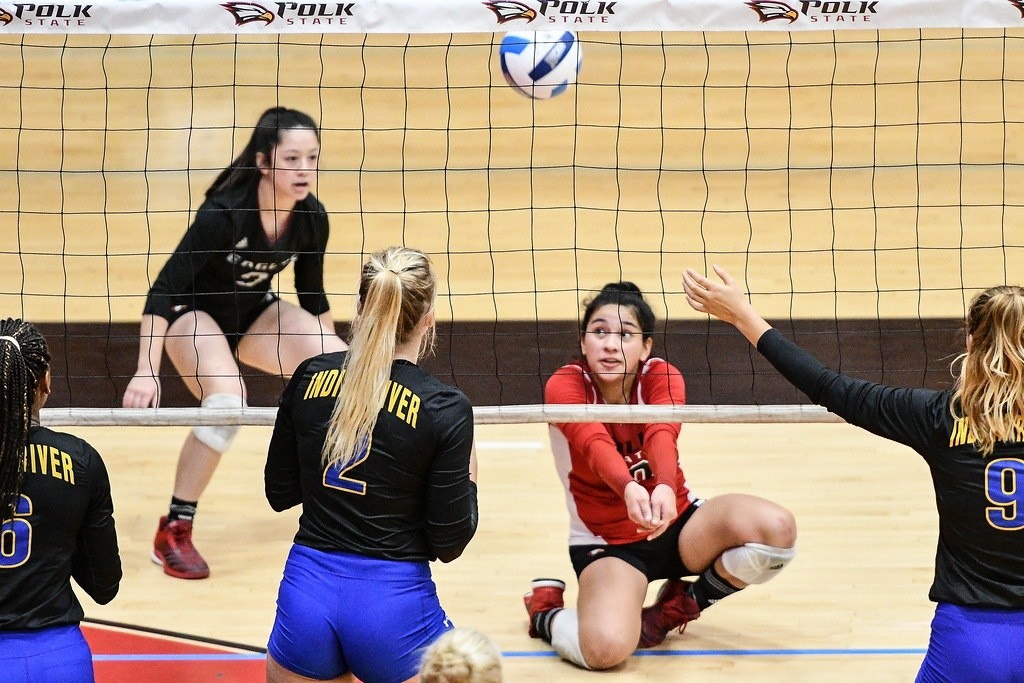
[123,106,348,579]
[418,625,504,683]
[524,282,797,670]
[0,317,123,683]
[265,246,478,683]
[682,264,1024,683]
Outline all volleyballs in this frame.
[497,30,584,102]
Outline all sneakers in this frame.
[151,516,209,578]
[637,578,701,648]
[524,579,565,639]
